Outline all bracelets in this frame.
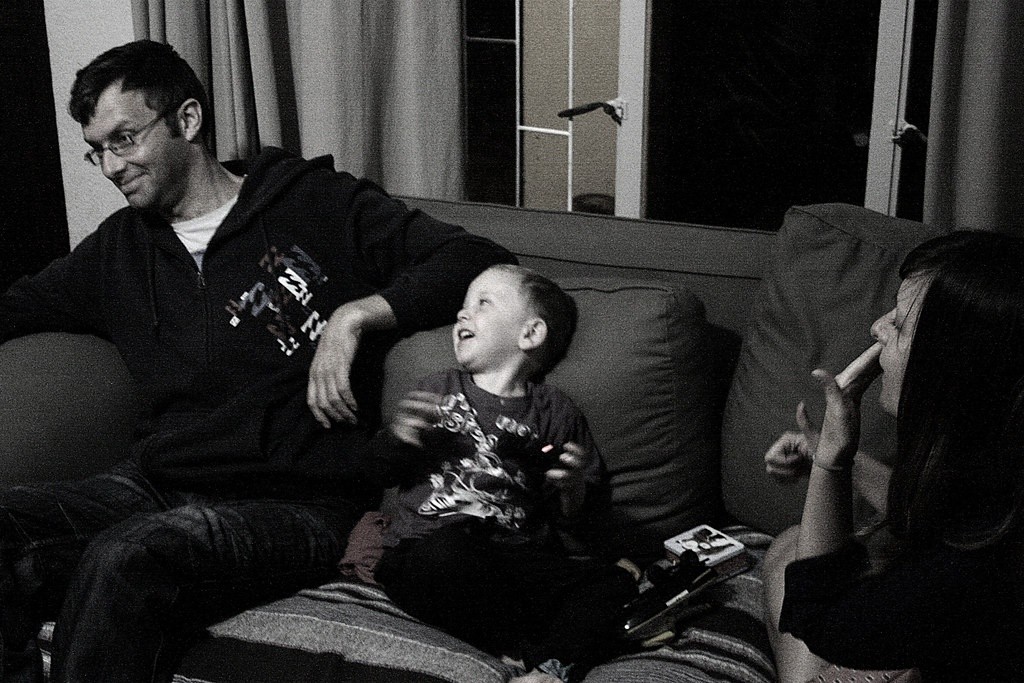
[812,453,855,471]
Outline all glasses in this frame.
[83,104,173,167]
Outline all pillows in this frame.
[383,278,704,516]
[719,204,947,545]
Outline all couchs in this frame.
[0,198,775,683]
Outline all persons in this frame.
[763,226,1024,683]
[362,262,637,683]
[1,37,521,683]
